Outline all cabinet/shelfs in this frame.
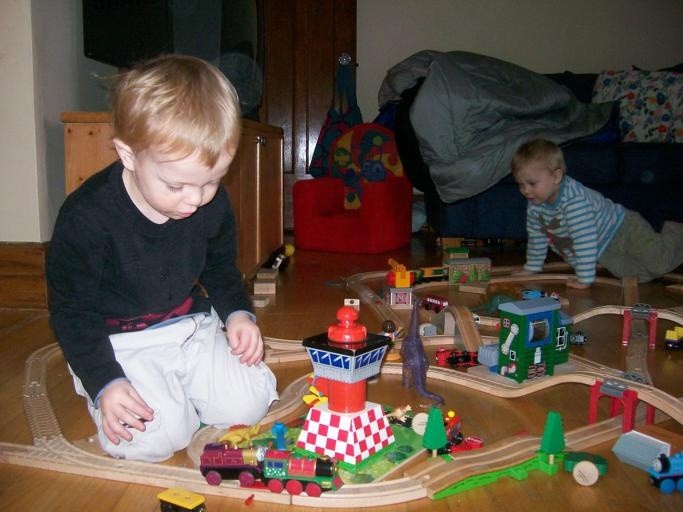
[57,111,284,289]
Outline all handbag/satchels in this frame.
[331,123,404,180]
[309,104,363,178]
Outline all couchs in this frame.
[291,123,413,255]
[426,61,683,245]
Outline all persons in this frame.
[509,138,682,290]
[43,53,277,462]
